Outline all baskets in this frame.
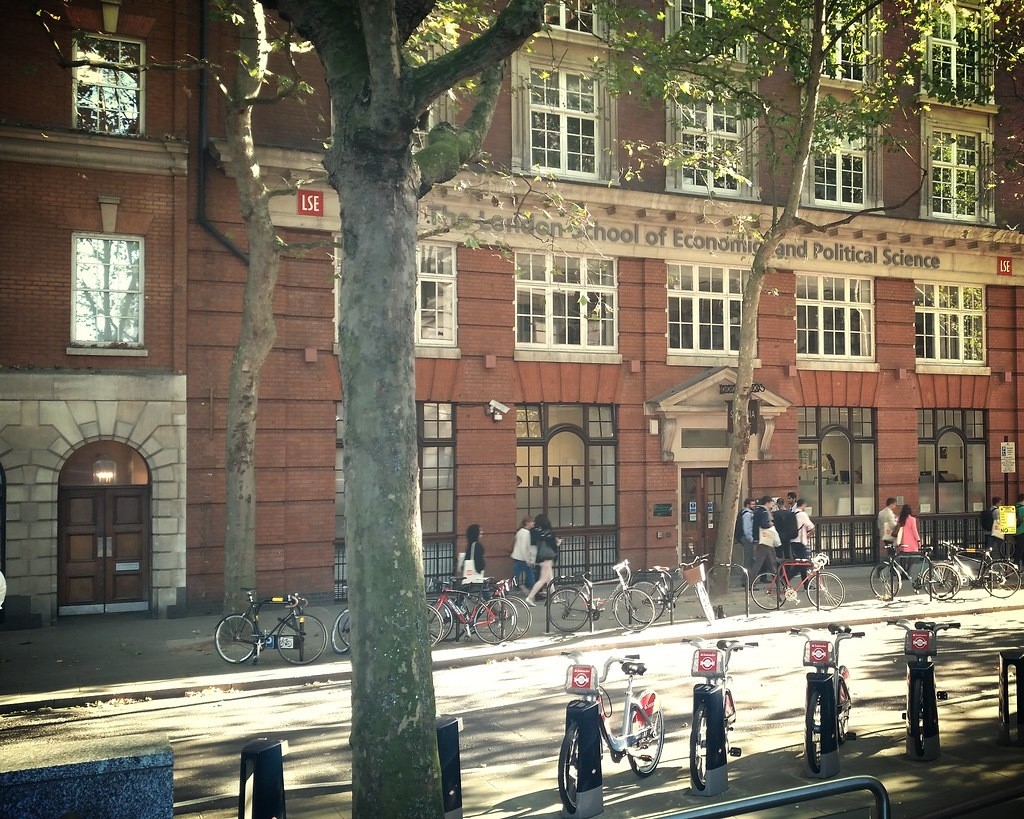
[681,563,706,585]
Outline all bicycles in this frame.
[931,541,1022,600]
[788,625,866,774]
[749,553,845,611]
[886,618,962,757]
[624,553,715,625]
[557,650,666,815]
[543,558,656,632]
[869,544,962,601]
[681,637,759,790]
[331,576,533,655]
[213,587,328,666]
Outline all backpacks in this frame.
[980,508,998,531]
[734,509,749,543]
[1016,505,1024,528]
[776,510,803,539]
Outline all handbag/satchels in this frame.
[462,542,485,585]
[991,519,1004,540]
[882,522,895,542]
[538,537,556,559]
[759,524,776,547]
[774,531,782,548]
[893,526,903,547]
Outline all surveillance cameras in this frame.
[489,398,510,414]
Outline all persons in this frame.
[741,498,755,586]
[511,516,547,601]
[1012,494,1024,573]
[897,504,921,580]
[985,497,1005,558]
[749,496,778,591]
[772,492,801,578]
[781,499,816,592]
[454,524,490,602]
[877,498,899,578]
[525,514,565,606]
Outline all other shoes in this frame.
[535,595,546,602]
[747,586,759,592]
[901,573,906,580]
[876,567,881,578]
[890,568,896,576]
[553,598,565,604]
[525,597,537,606]
[512,584,521,592]
[804,586,816,592]
[760,577,772,584]
